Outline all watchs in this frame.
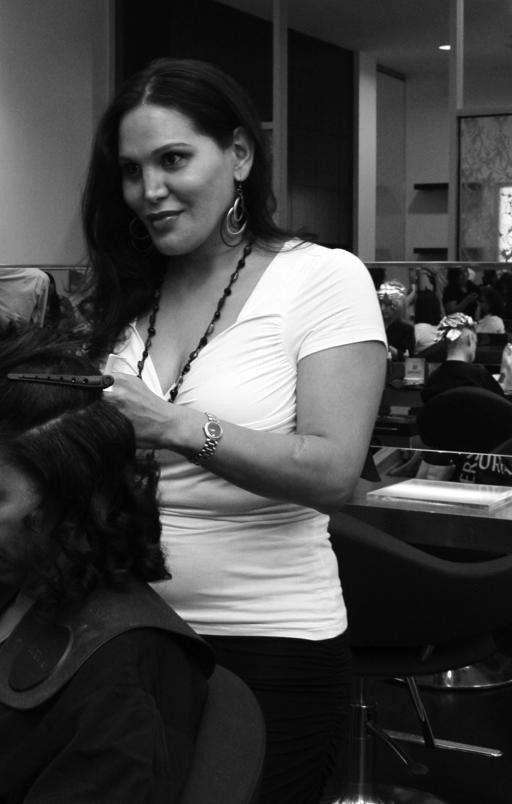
[188,412,223,465]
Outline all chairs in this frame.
[315,509,511,804]
[416,388,511,459]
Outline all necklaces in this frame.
[136,230,258,484]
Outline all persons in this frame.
[0,319,216,804]
[377,264,512,405]
[82,59,389,804]
